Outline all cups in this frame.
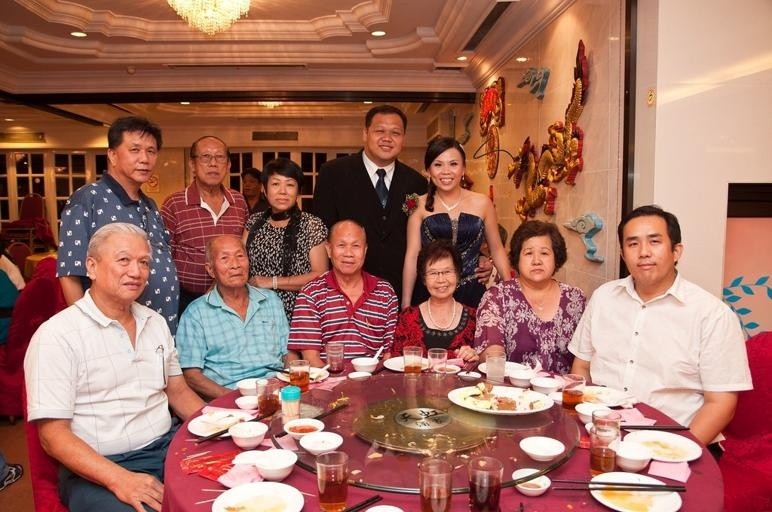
[560,372,586,413]
[288,359,312,394]
[484,350,507,385]
[426,347,450,375]
[591,408,623,432]
[313,450,352,512]
[467,452,505,511]
[587,422,619,479]
[255,376,282,415]
[401,344,425,374]
[417,455,455,511]
[325,341,346,376]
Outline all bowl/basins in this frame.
[607,440,654,475]
[508,369,533,389]
[252,447,300,481]
[233,375,268,395]
[573,400,615,423]
[300,428,345,456]
[518,433,568,463]
[229,420,271,453]
[510,466,553,497]
[284,416,328,440]
[274,365,330,385]
[346,355,381,380]
[530,375,560,395]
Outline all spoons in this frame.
[310,363,331,382]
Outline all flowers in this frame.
[402,192,419,217]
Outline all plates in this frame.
[210,479,306,512]
[587,470,684,511]
[188,410,275,442]
[382,352,434,372]
[458,371,482,380]
[578,383,630,406]
[620,426,704,465]
[234,395,260,410]
[549,390,563,405]
[435,365,461,374]
[478,360,532,379]
[447,384,555,417]
[348,370,373,381]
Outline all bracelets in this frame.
[271,275,278,290]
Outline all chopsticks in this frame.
[195,411,276,444]
[262,360,314,381]
[274,403,350,438]
[551,477,685,493]
[335,493,385,511]
[619,424,691,431]
[464,361,480,374]
[371,367,388,376]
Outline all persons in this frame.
[285,218,398,369]
[0,232,26,346]
[24,221,209,511]
[472,219,588,374]
[567,205,754,464]
[159,135,250,322]
[240,155,330,326]
[399,136,512,310]
[390,239,478,362]
[54,116,180,348]
[309,104,493,313]
[174,234,302,399]
[240,167,270,216]
[473,223,507,288]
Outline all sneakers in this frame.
[0,465,23,491]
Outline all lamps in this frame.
[166,0,251,37]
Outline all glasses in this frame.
[193,151,226,163]
[156,345,166,384]
[423,269,457,279]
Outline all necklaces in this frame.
[426,295,456,329]
[523,284,552,311]
[434,186,462,212]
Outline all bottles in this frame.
[279,385,302,425]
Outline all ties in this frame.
[375,169,389,208]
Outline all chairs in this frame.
[716,331,771,512]
[0,191,69,424]
[22,381,70,512]
